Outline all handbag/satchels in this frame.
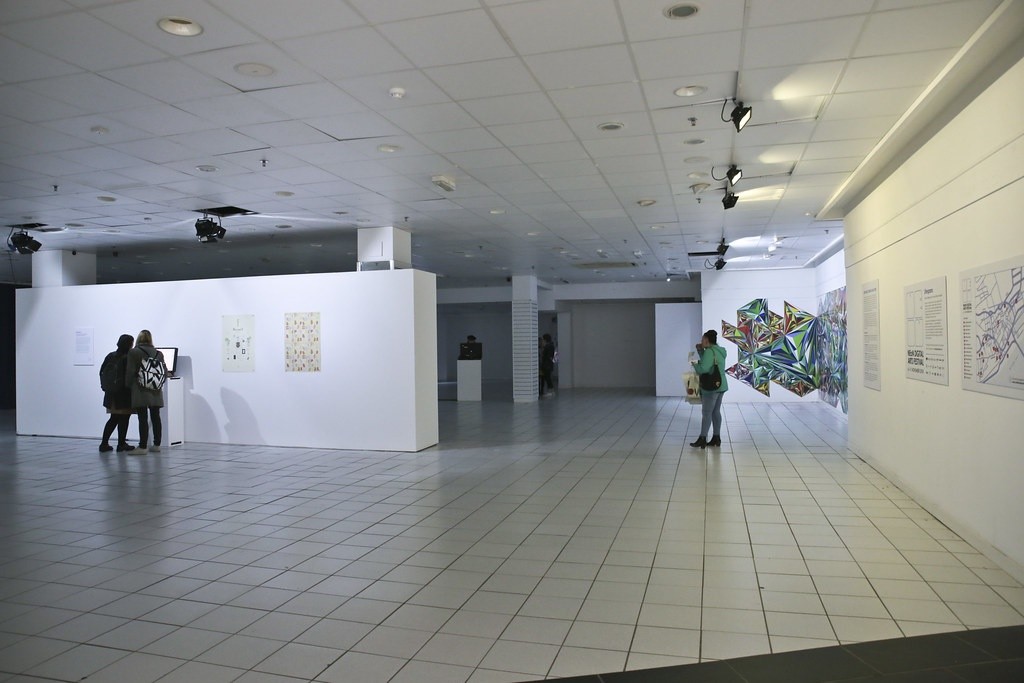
[699,364,722,392]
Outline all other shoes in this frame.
[99,445,113,452]
[117,442,135,452]
[542,392,555,400]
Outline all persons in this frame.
[457,336,476,360]
[690,330,729,448]
[99,334,137,452]
[125,330,167,454]
[540,334,556,397]
[538,337,544,389]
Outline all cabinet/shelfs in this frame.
[456,360,481,402]
[147,377,184,447]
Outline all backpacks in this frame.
[101,352,128,393]
[135,346,167,393]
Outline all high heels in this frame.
[690,436,706,449]
[706,435,721,447]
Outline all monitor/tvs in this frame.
[156,347,178,374]
[460,343,482,359]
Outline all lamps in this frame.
[721,98,752,133]
[717,238,729,256]
[710,165,742,187]
[715,258,727,270]
[11,231,42,255]
[692,185,705,194]
[432,176,457,192]
[195,218,226,243]
[721,192,739,209]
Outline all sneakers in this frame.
[128,447,148,455]
[149,446,161,452]
